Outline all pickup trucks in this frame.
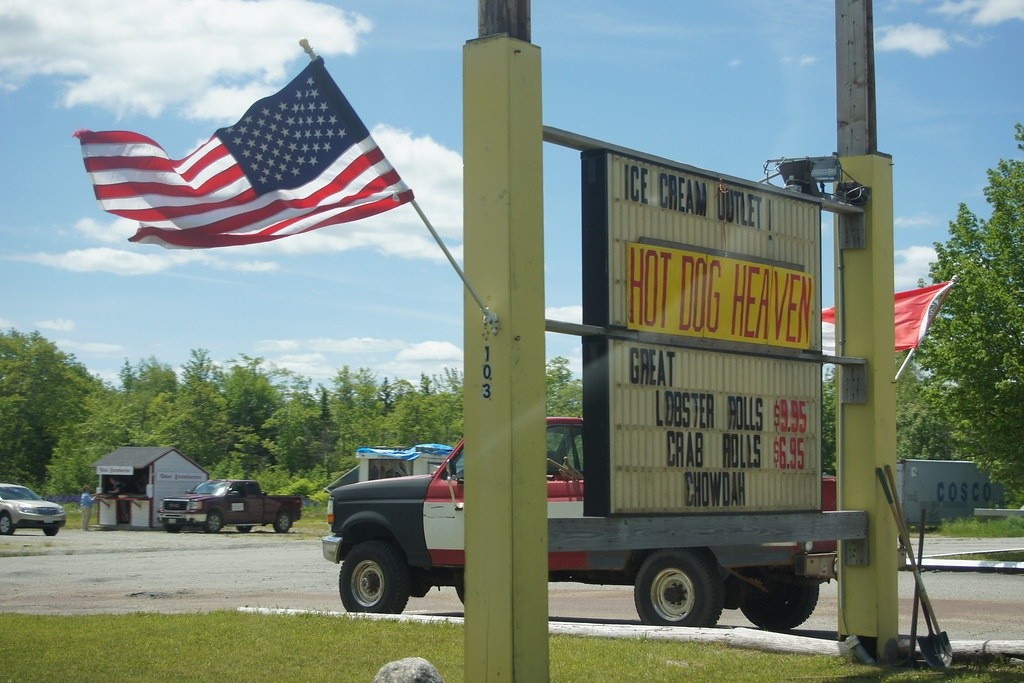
[157,478,300,535]
[325,412,836,627]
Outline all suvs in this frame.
[0,482,69,538]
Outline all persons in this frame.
[107,476,129,522]
[79,485,96,531]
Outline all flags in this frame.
[821,280,953,352]
[74,57,415,250]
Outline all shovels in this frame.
[885,464,952,670]
